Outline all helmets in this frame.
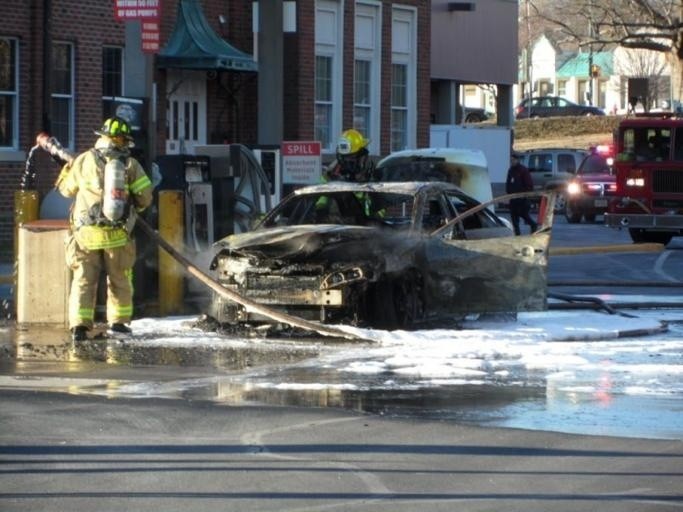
[337,129,370,154]
[94,115,133,140]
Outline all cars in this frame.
[205,177,558,326]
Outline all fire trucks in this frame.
[602,108,683,248]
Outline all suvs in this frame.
[563,144,618,223]
[514,95,608,117]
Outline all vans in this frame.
[519,146,588,216]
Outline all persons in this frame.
[505,154,537,236]
[315,129,387,223]
[55,117,155,342]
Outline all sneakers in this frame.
[111,321,132,334]
[73,325,89,343]
